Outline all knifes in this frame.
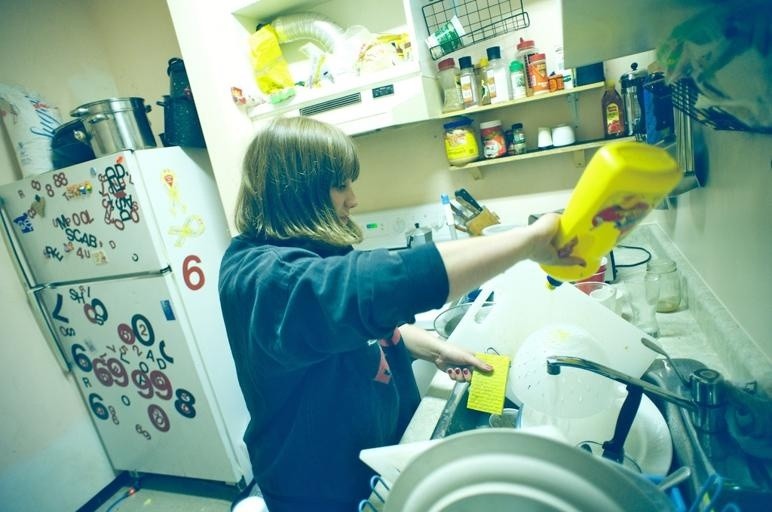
[451,189,482,234]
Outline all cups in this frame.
[624,274,664,340]
[572,281,617,312]
[537,126,551,149]
[423,16,469,59]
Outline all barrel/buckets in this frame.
[541,143,682,290]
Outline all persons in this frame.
[217,115,587,512]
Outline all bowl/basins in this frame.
[552,123,575,147]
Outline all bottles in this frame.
[455,56,479,107]
[435,58,464,113]
[600,79,627,139]
[477,55,496,106]
[508,59,529,100]
[643,257,682,314]
[528,52,552,98]
[442,118,530,169]
[483,44,510,103]
[515,35,539,97]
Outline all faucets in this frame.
[548,337,723,409]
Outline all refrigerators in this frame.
[1,143,260,505]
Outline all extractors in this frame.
[243,14,429,156]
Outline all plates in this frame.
[378,426,680,512]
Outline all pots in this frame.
[404,222,432,248]
[70,96,159,159]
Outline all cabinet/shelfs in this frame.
[438,79,637,171]
